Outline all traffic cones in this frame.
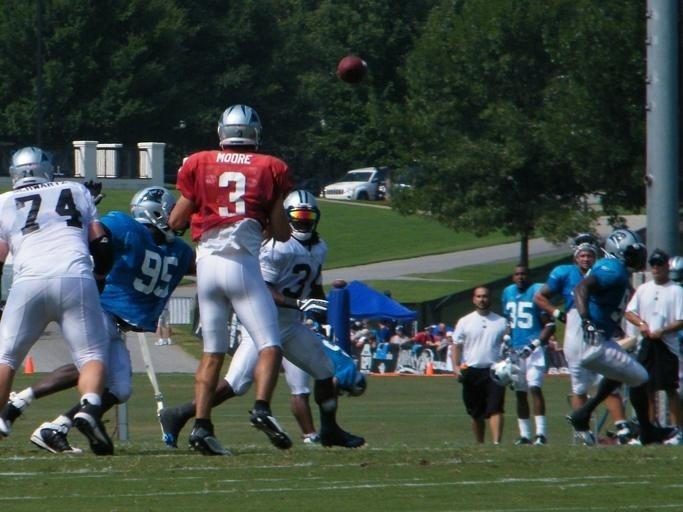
[423,361,434,376]
[22,356,35,374]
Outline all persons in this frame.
[167,104,292,455]
[154,304,171,345]
[159,189,365,448]
[0,147,113,455]
[4,179,196,453]
[282,334,366,443]
[303,318,448,373]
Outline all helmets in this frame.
[216,105,264,151]
[8,146,53,189]
[129,186,191,236]
[350,372,368,397]
[281,190,321,241]
[571,230,683,280]
[490,360,512,388]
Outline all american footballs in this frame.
[339,56,365,83]
[599,433,637,446]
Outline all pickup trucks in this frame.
[317,165,411,202]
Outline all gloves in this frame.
[498,339,531,366]
[296,297,331,312]
[581,318,606,345]
[83,179,105,205]
[558,312,567,323]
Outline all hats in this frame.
[395,325,404,333]
[349,317,363,331]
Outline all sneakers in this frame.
[249,407,293,449]
[302,428,365,448]
[0,391,116,457]
[514,435,547,448]
[159,405,228,456]
[154,338,171,346]
[563,408,683,450]
